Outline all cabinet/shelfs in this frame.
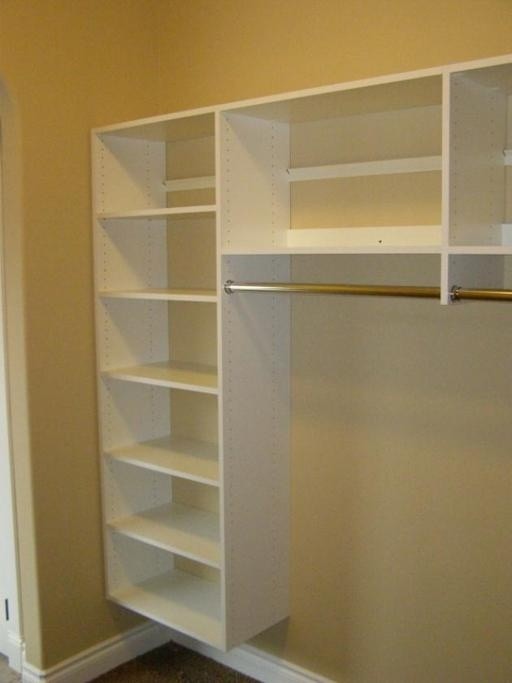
[89,56,512,683]
